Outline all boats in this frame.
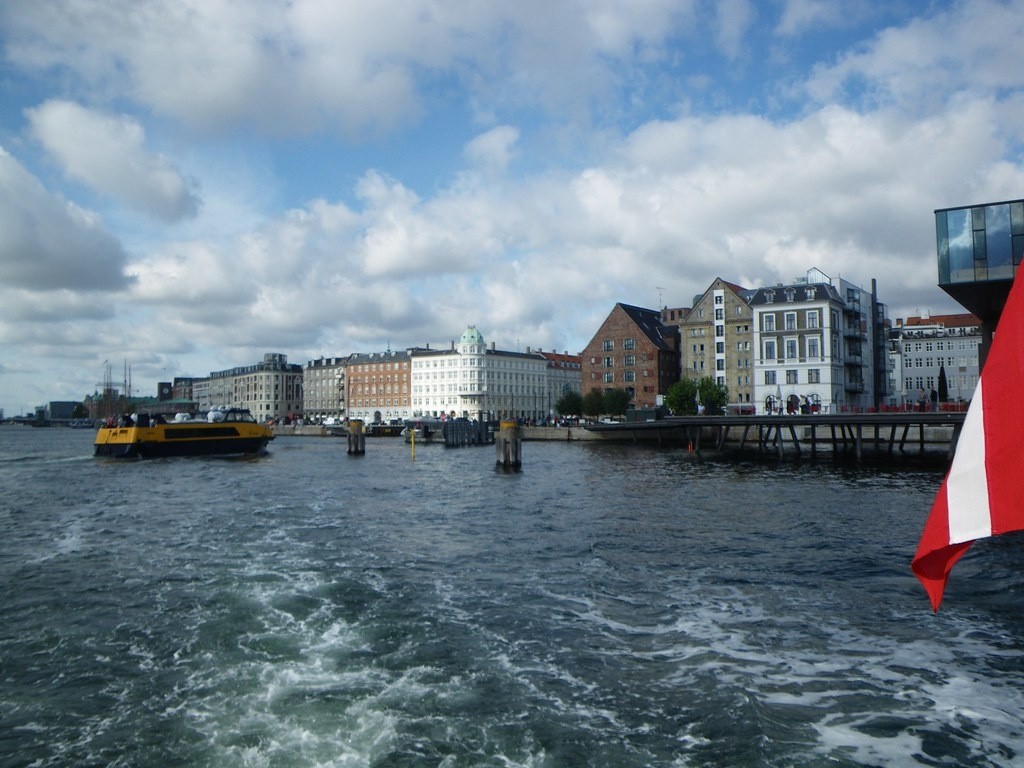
[93,406,278,458]
[399,418,474,444]
[71,418,95,428]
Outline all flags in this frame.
[912,259,1024,612]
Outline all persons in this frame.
[108,411,137,428]
[918,386,939,413]
[500,414,574,427]
[766,395,815,414]
[267,415,304,426]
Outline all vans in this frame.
[322,416,341,426]
